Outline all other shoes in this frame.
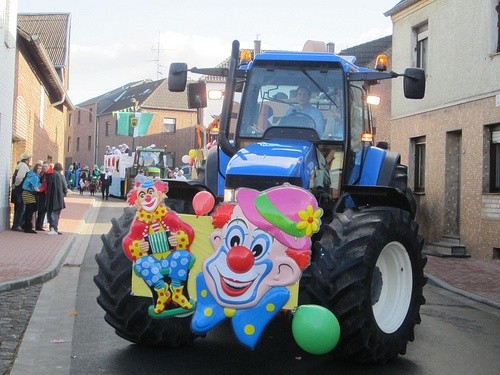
[57,232,62,235]
[12,226,24,231]
[24,230,37,233]
[36,228,46,231]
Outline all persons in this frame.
[276,86,324,140]
[34,160,66,235]
[53,163,68,235]
[66,143,187,200]
[22,163,42,233]
[11,151,33,231]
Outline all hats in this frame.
[20,150,32,159]
[53,163,63,170]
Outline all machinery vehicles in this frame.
[94,40,428,358]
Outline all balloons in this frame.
[192,191,215,215]
[291,304,341,355]
[189,149,198,158]
[182,155,191,163]
